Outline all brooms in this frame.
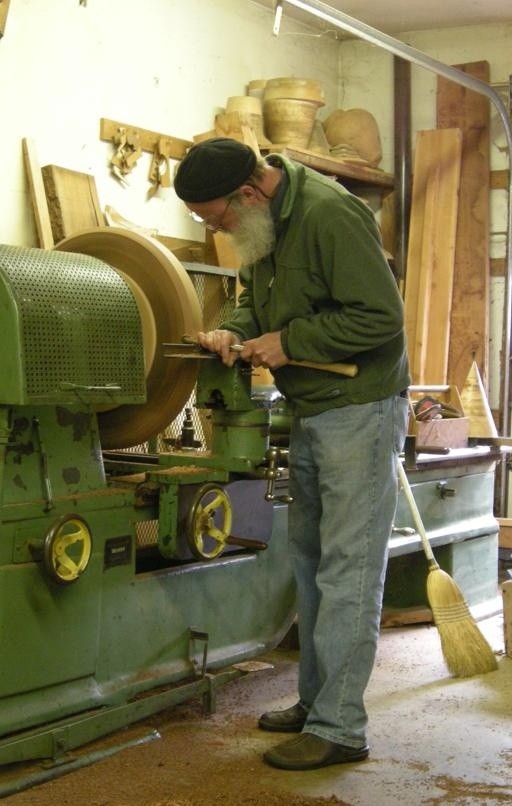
[394,457,498,677]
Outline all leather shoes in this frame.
[258,698,311,732]
[261,728,370,771]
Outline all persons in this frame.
[172,135,410,771]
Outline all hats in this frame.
[173,135,259,203]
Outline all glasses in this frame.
[188,197,236,232]
